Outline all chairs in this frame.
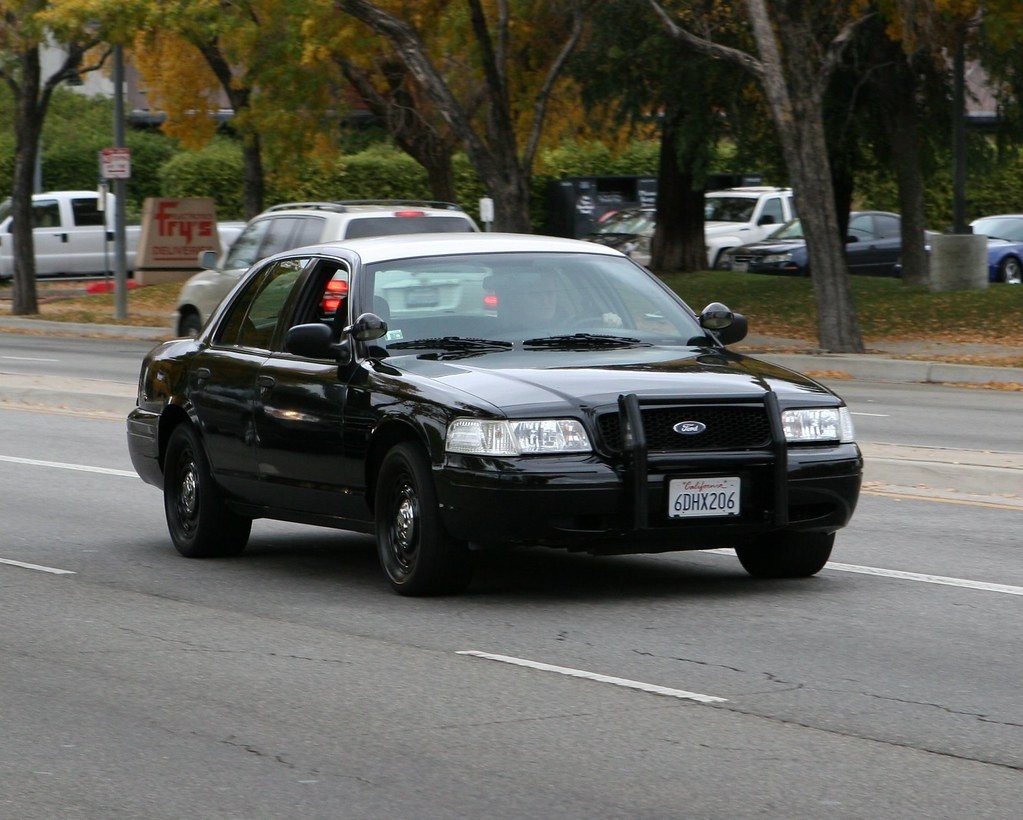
[332,296,390,344]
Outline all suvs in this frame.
[174,198,501,357]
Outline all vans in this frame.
[702,186,800,269]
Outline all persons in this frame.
[495,262,622,332]
[31,207,53,227]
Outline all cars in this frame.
[578,207,658,269]
[970,215,1023,286]
[719,209,947,279]
[127,231,865,599]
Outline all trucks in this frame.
[0,188,248,285]
